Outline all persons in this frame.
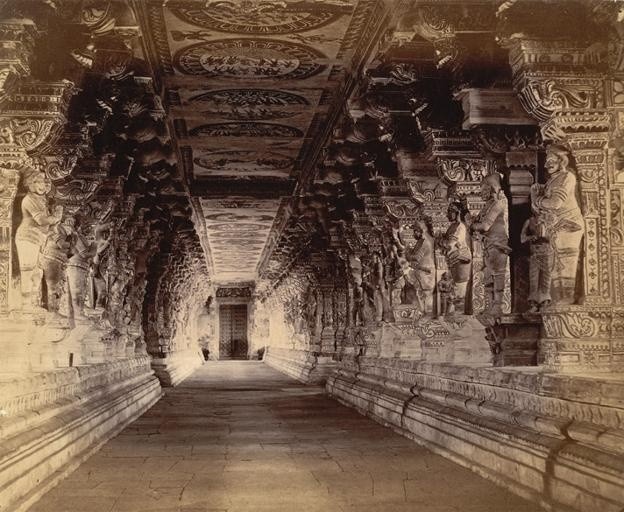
[347,221,435,326]
[435,201,471,312]
[464,173,511,317]
[41,212,146,323]
[530,148,583,311]
[15,173,65,305]
